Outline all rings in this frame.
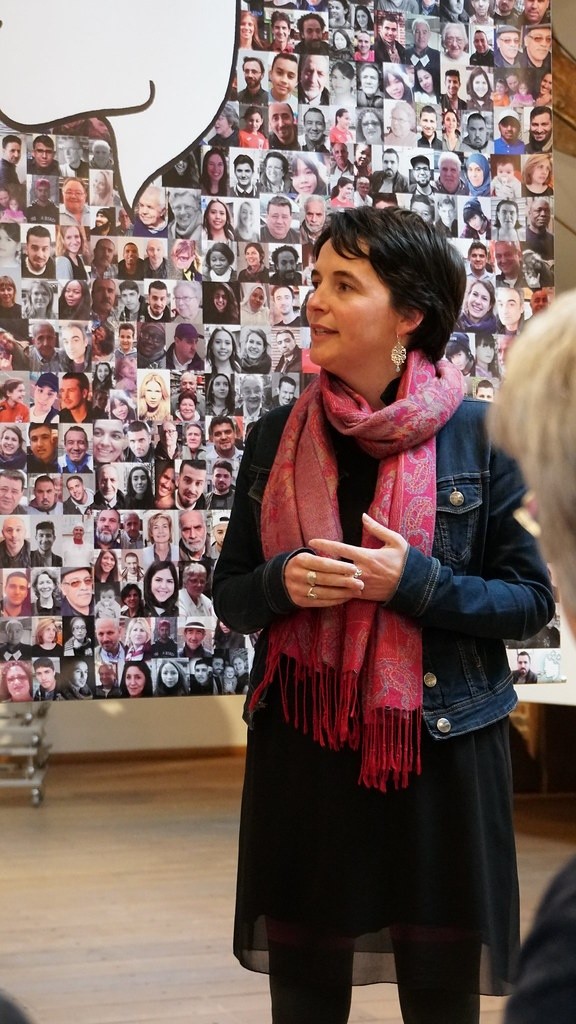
[353,570,363,578]
[307,569,317,586]
[307,587,318,600]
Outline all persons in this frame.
[331,60,357,106]
[436,152,468,195]
[372,148,407,192]
[268,53,299,103]
[413,65,438,103]
[467,69,494,110]
[213,208,553,1024]
[0,0,560,704]
[483,285,576,1024]
[299,107,330,152]
[330,144,353,174]
[461,113,494,154]
[239,105,268,150]
[385,102,417,146]
[200,148,230,196]
[261,196,300,243]
[132,186,168,237]
